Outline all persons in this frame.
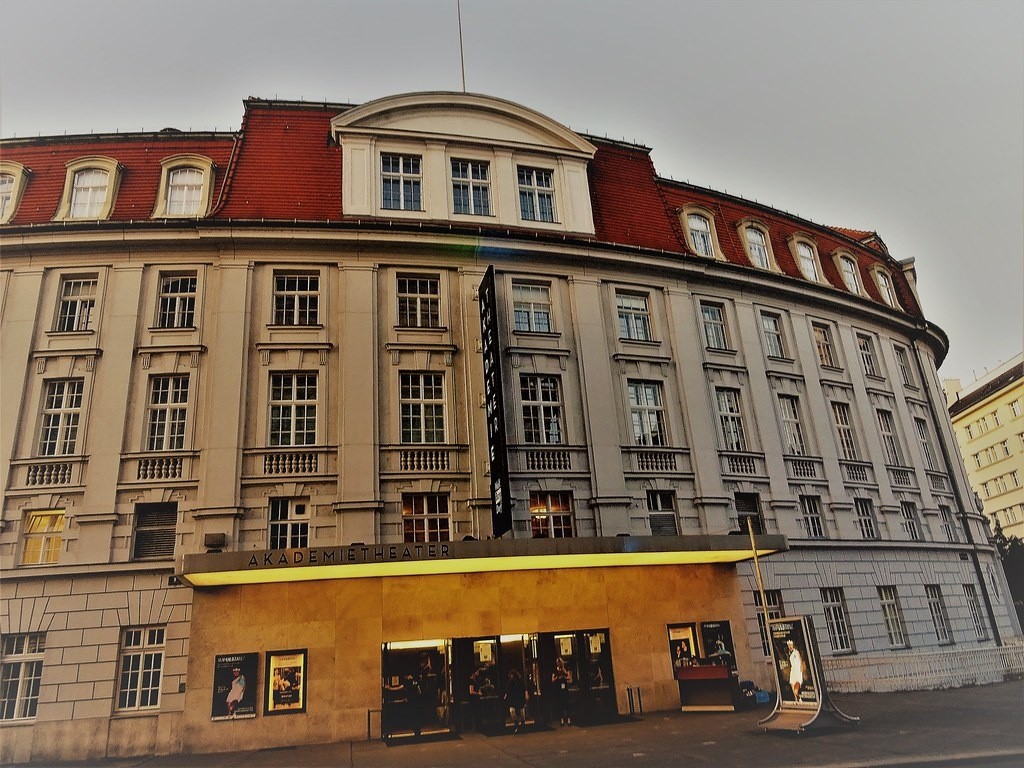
[785,637,804,707]
[273,667,300,691]
[691,656,701,667]
[465,667,486,732]
[226,667,246,719]
[551,657,572,727]
[503,669,530,736]
[676,641,689,659]
[383,669,423,738]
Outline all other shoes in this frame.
[514,725,519,733]
[561,719,564,727]
[567,718,571,727]
